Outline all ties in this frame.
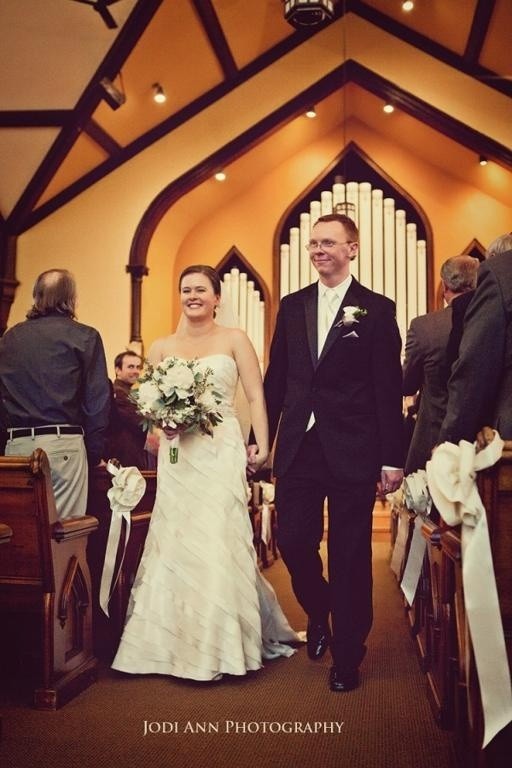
[303,288,339,433]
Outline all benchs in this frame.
[0,448,102,711]
[107,466,161,681]
[391,435,511,768]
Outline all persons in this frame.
[110,350,148,469]
[439,232,512,385]
[110,265,307,682]
[399,255,478,481]
[248,213,403,692]
[1,269,112,525]
[432,250,512,455]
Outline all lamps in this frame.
[153,83,166,102]
[384,102,393,113]
[306,106,317,118]
[479,155,489,167]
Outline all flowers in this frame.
[127,355,224,466]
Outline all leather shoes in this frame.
[326,656,360,692]
[304,615,331,660]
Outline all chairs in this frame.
[247,466,279,570]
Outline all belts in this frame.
[4,426,83,441]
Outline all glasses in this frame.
[304,239,351,250]
